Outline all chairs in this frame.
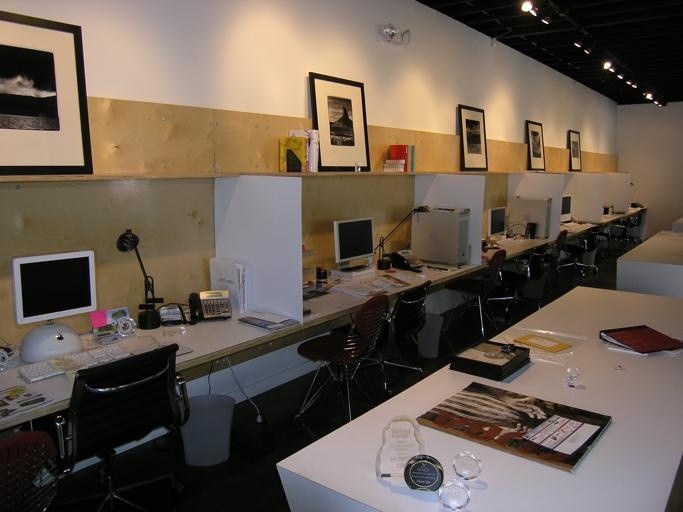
[354,277,431,395]
[32,344,191,511]
[293,294,392,426]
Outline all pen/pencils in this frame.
[427,265,448,271]
[316,260,328,272]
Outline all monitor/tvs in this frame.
[333,217,375,273]
[11,250,98,363]
[487,206,507,238]
[561,195,572,222]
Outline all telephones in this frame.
[188,289,232,322]
[391,250,424,270]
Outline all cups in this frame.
[354,162,360,173]
[438,451,484,511]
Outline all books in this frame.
[238,312,298,331]
[416,381,612,474]
[599,323,682,357]
[209,256,247,314]
[279,128,320,174]
[384,144,408,173]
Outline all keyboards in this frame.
[17,343,132,382]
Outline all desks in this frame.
[476,205,647,341]
[616,229,680,295]
[1,249,473,432]
[279,285,682,512]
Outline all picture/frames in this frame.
[307,69,371,173]
[524,120,546,172]
[457,103,490,171]
[567,129,582,172]
[0,8,95,175]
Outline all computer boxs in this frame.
[411,206,472,267]
[507,195,553,239]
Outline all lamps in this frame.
[520,0,665,109]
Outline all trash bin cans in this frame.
[180,394,235,467]
[581,248,598,273]
[405,313,444,358]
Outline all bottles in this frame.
[608,204,614,216]
[565,352,580,387]
[308,130,319,173]
